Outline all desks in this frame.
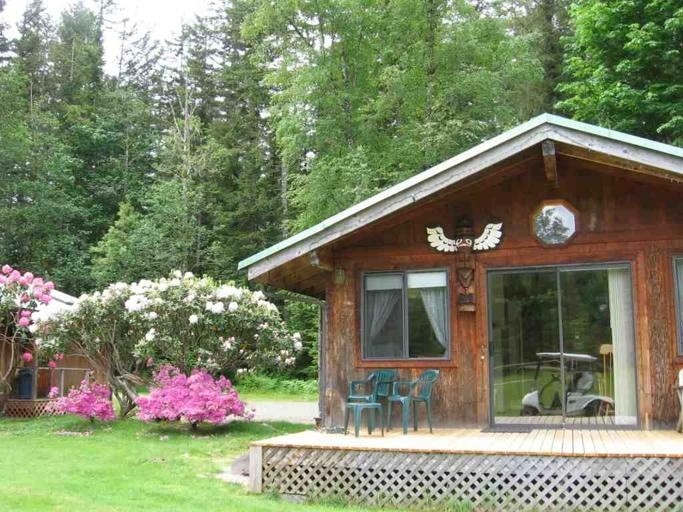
[344,402,384,438]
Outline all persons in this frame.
[575,366,594,395]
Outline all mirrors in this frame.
[528,199,580,249]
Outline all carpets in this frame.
[480,425,533,432]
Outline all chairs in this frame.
[386,368,440,435]
[347,368,399,432]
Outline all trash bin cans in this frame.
[15,367,33,399]
